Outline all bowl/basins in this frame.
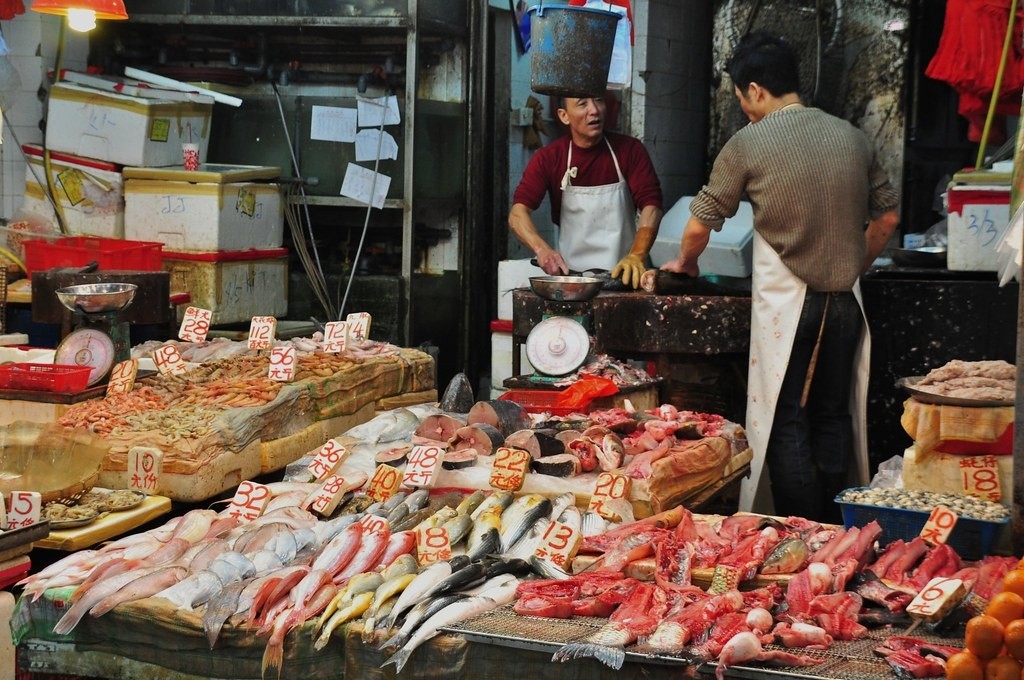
[53,284,140,314]
[528,273,606,303]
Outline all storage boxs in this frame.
[22,236,166,282]
[21,143,126,242]
[123,162,285,254]
[490,257,553,403]
[160,249,289,325]
[44,72,214,167]
[947,186,1014,269]
[0,348,436,503]
[650,192,756,278]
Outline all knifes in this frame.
[529,259,582,274]
[58,262,98,277]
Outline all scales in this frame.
[52,282,138,388]
[502,276,605,393]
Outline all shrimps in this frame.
[60,352,354,443]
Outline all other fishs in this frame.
[271,331,413,368]
[130,336,250,360]
[13,400,1018,680]
[590,268,752,296]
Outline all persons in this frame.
[660,32,903,527]
[507,98,662,289]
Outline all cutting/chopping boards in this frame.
[509,285,595,343]
[30,270,173,324]
[590,293,753,357]
